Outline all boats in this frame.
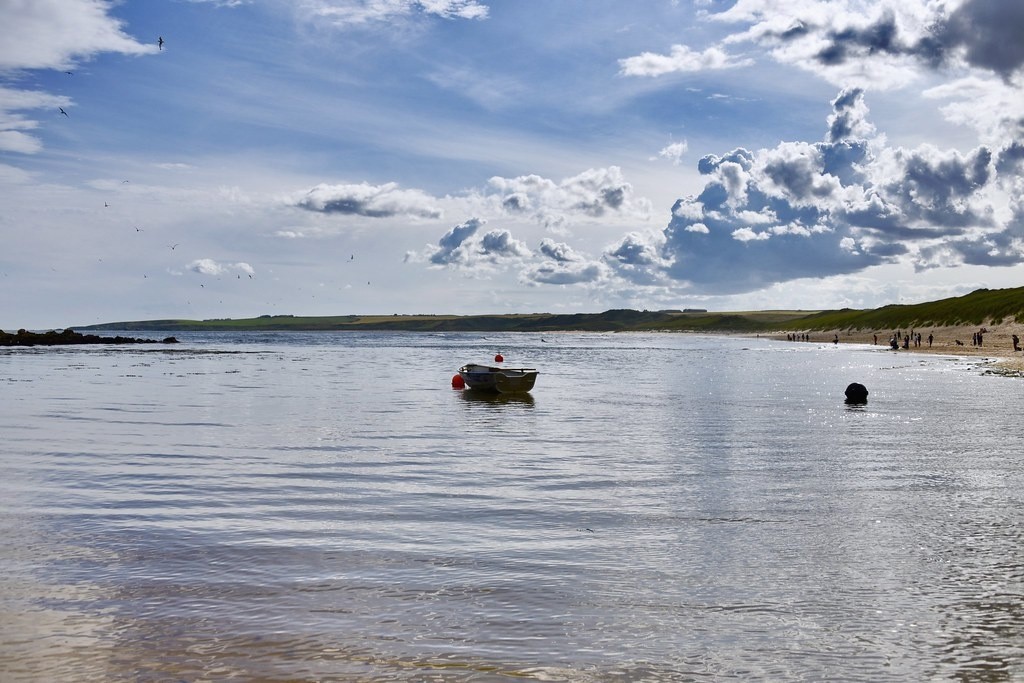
[457,362,540,397]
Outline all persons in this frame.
[1011,334,1019,351]
[927,333,935,347]
[834,334,838,341]
[972,328,986,347]
[786,332,810,342]
[873,334,877,345]
[888,327,922,350]
